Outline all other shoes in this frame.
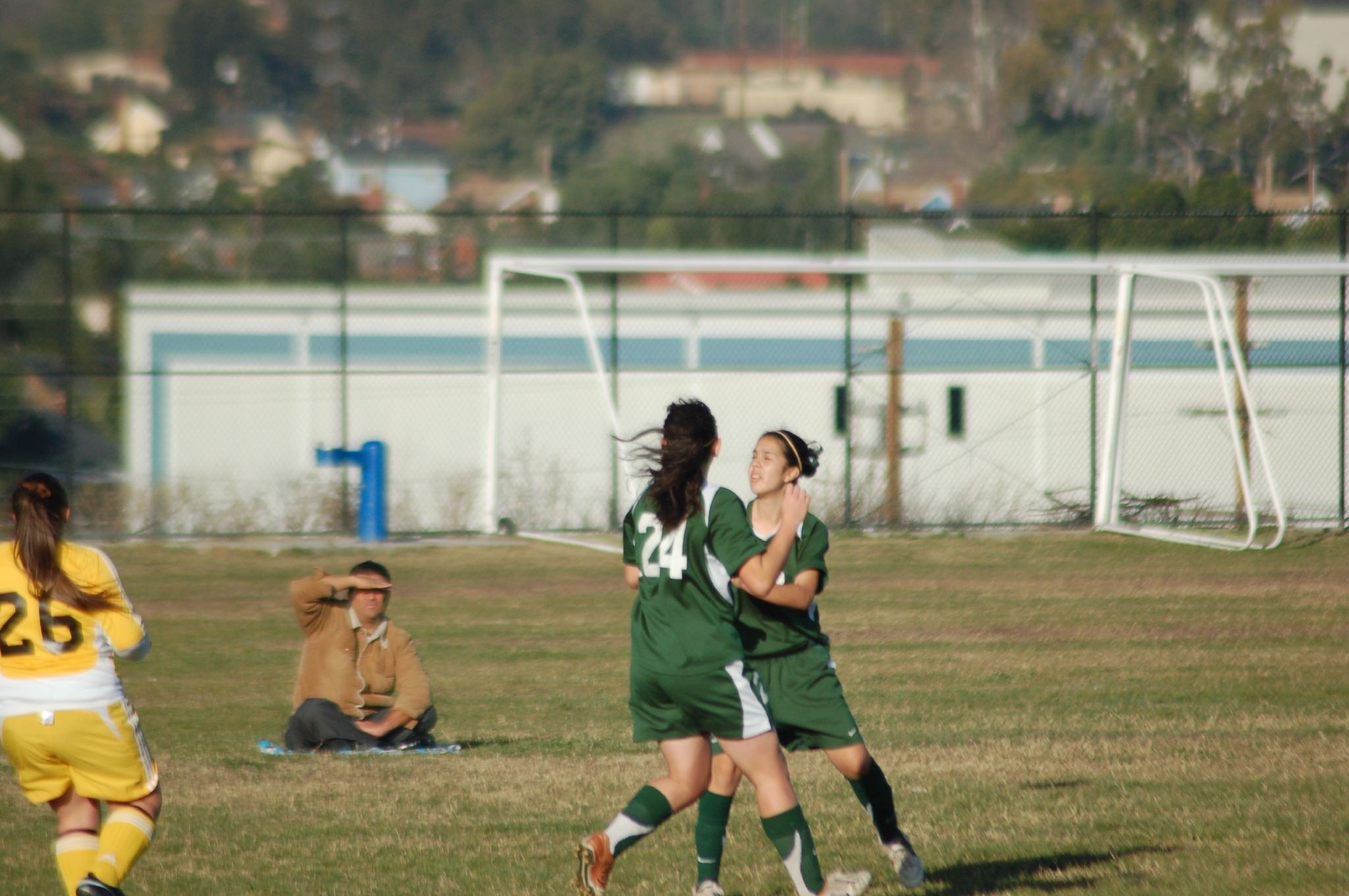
[413,731,437,749]
[75,873,122,896]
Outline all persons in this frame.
[282,560,435,751]
[0,469,161,896]
[694,431,923,896]
[569,400,870,896]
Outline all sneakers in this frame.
[881,836,925,889]
[577,832,614,896]
[691,878,725,896]
[817,869,871,896]
